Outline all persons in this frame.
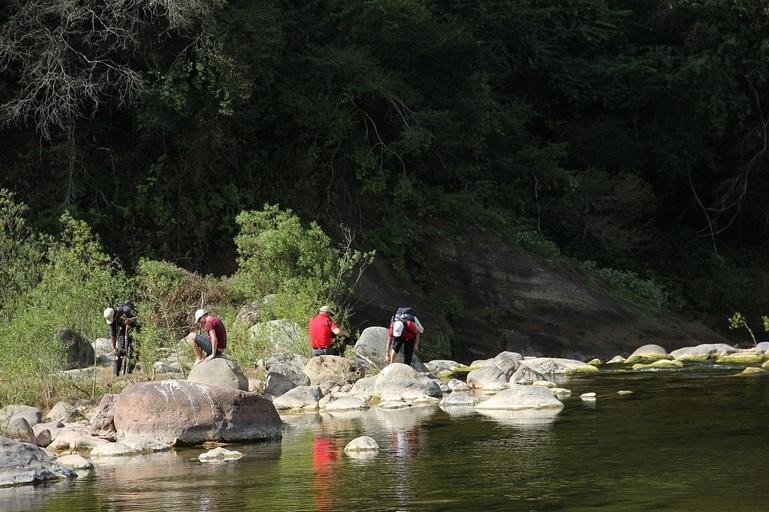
[308,306,348,356]
[187,308,227,367]
[385,320,421,365]
[103,306,141,378]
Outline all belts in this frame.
[314,347,328,350]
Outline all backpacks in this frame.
[119,302,137,317]
[393,308,418,320]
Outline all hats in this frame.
[320,306,335,316]
[103,308,115,324]
[393,321,404,337]
[194,309,207,324]
